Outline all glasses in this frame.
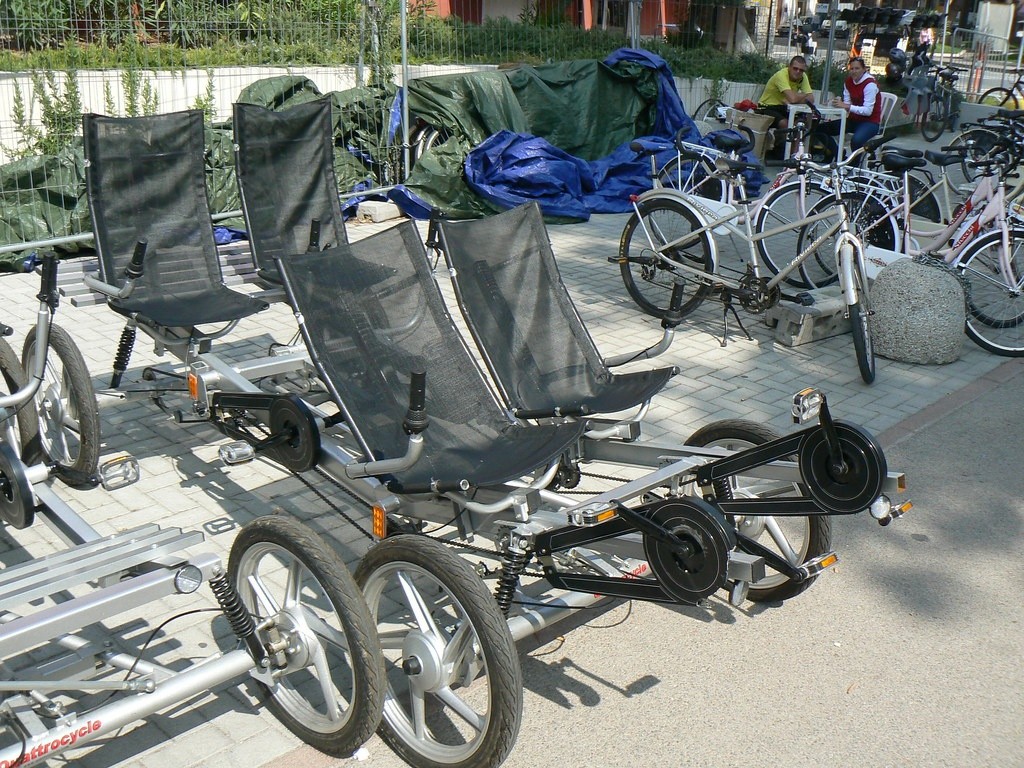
[790,65,805,73]
[854,57,864,68]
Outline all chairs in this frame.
[428,202,681,442]
[231,92,398,300]
[832,91,898,168]
[270,219,594,512]
[81,106,270,353]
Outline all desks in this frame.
[782,104,846,172]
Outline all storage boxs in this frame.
[725,109,775,165]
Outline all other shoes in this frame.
[835,149,846,162]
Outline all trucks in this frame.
[821,2,856,38]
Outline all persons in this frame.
[817,57,881,168]
[758,56,814,127]
[920,22,934,47]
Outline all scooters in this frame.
[886,42,930,84]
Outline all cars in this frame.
[777,16,819,37]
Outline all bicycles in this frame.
[35,89,912,768]
[602,108,1024,385]
[977,69,1024,110]
[920,64,969,143]
[0,250,388,768]
